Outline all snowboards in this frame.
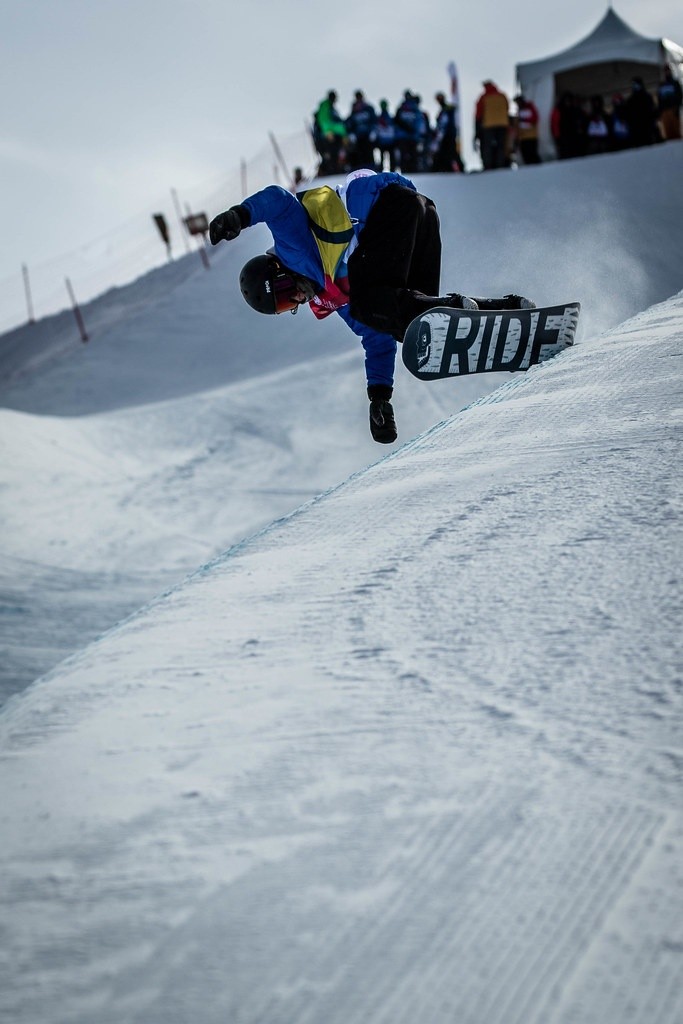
[401,301,581,381]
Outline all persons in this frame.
[309,62,683,177]
[209,168,536,444]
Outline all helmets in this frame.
[239,256,316,315]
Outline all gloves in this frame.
[367,385,398,443]
[207,205,252,244]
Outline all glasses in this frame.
[273,277,298,313]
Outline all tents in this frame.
[516,7,682,164]
